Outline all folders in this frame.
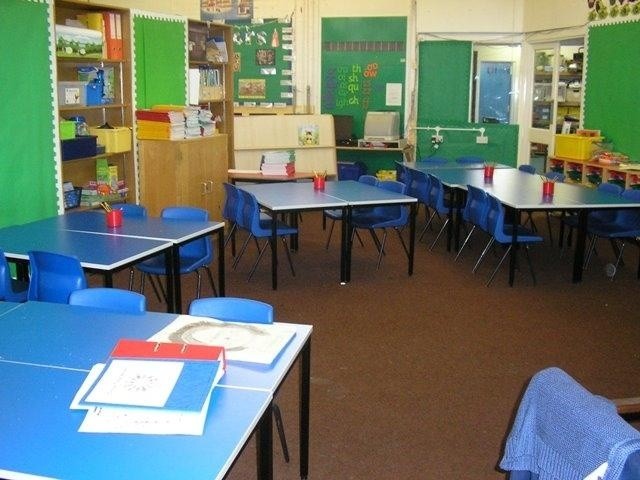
[102,11,124,60]
[78,338,227,416]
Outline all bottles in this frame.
[69,116,89,136]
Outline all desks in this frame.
[0,299,317,480]
[335,146,410,160]
[0,209,224,316]
[243,175,419,293]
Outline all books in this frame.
[187,37,229,105]
[260,149,296,177]
[297,123,320,148]
[135,103,217,142]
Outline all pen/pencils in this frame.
[100,200,113,212]
[482,159,496,166]
[540,174,559,182]
[312,169,327,177]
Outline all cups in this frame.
[107,209,122,228]
[314,176,325,191]
[485,167,493,177]
[543,182,554,195]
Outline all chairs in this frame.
[496,363,638,480]
[318,172,381,251]
[185,294,294,466]
[230,190,302,283]
[393,158,638,289]
[22,249,88,307]
[0,251,30,303]
[219,180,272,263]
[104,201,163,304]
[66,287,149,316]
[134,205,219,307]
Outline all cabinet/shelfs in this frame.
[0,0,135,213]
[530,70,582,156]
[549,155,640,185]
[186,19,235,171]
[137,134,227,241]
